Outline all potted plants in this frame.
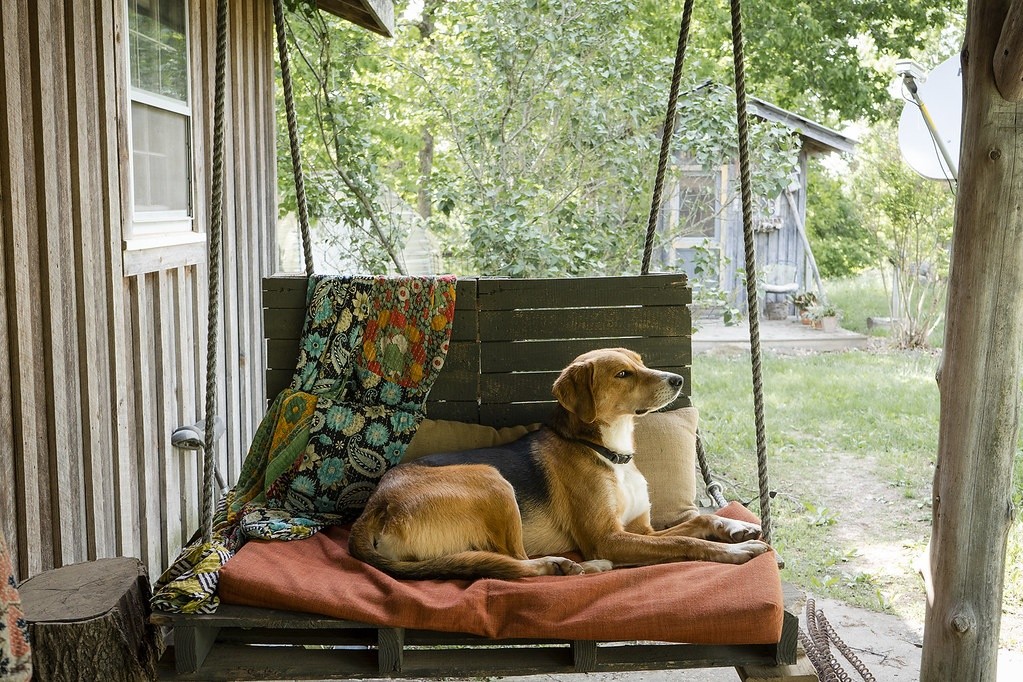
[791,291,845,332]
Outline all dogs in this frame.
[345,348,772,580]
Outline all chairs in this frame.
[760,258,801,317]
